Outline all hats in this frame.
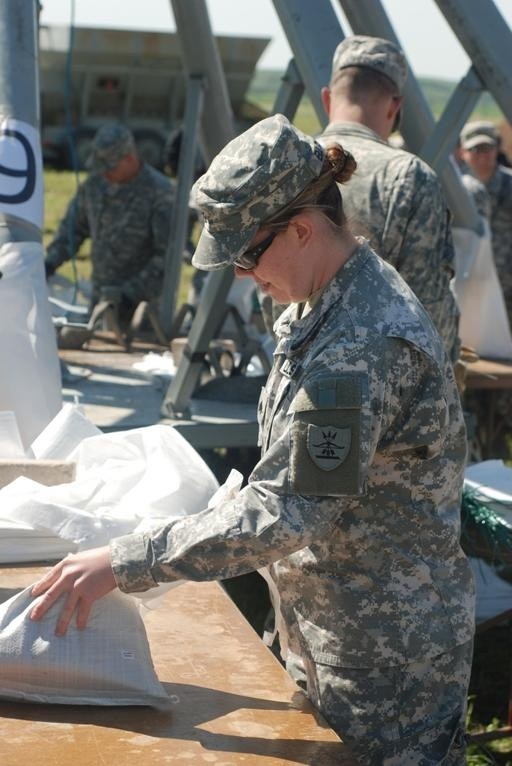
[187,113,326,272]
[84,124,134,174]
[459,120,498,151]
[332,36,408,133]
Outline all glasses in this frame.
[235,233,276,271]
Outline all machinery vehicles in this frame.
[39,26,272,176]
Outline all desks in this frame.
[464,358,512,459]
[0,563,359,766]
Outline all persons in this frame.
[31,116,475,766]
[315,34,462,372]
[448,122,512,444]
[39,120,176,332]
